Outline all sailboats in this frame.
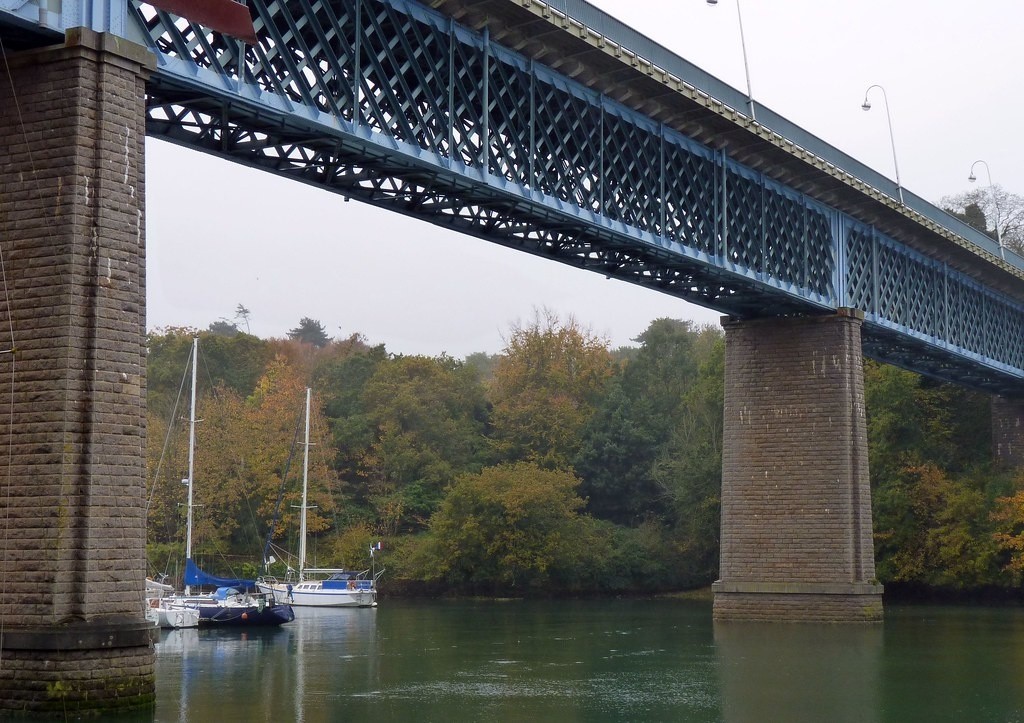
[144,340,298,629]
[252,385,381,609]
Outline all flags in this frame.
[374,542,384,551]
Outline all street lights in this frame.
[968,159,1006,261]
[708,0,758,120]
[861,83,904,203]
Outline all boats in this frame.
[146,558,173,601]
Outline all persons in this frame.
[350,581,356,591]
